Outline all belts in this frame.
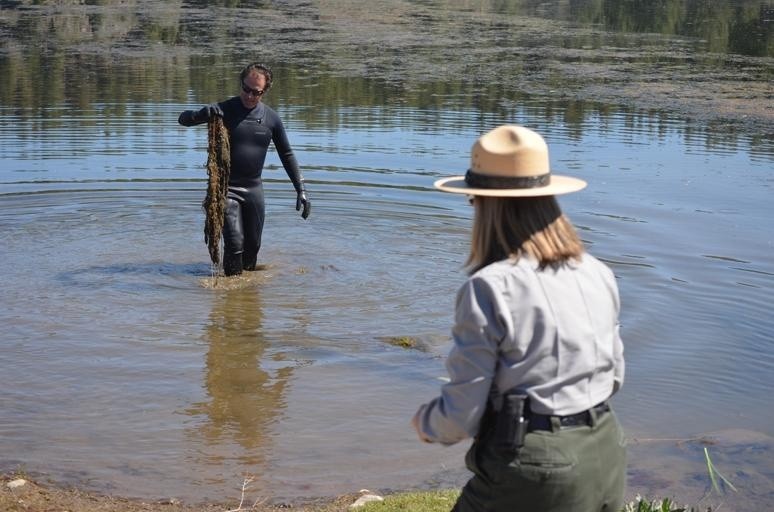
[530,406,607,429]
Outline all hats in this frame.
[433,124,587,197]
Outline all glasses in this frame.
[241,79,264,96]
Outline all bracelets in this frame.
[298,190,305,193]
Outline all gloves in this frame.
[296,191,311,220]
[193,105,224,121]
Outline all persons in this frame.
[411,124,625,512]
[178,63,311,275]
